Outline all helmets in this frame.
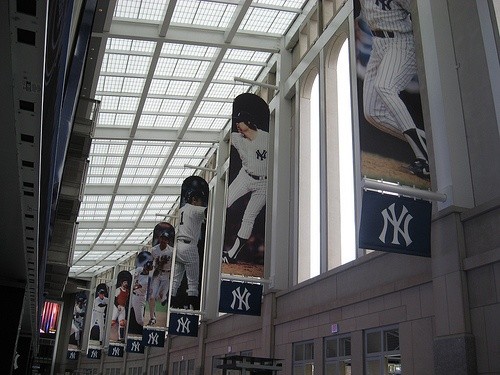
[98,289,105,294]
[185,188,205,197]
[160,231,171,240]
[233,111,256,122]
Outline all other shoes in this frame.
[160,300,176,306]
[221,250,236,264]
[148,313,156,326]
[118,337,125,343]
[99,341,102,345]
[407,160,430,182]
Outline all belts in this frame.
[244,169,267,180]
[162,270,170,272]
[183,240,191,244]
[74,320,79,323]
[133,291,139,296]
[370,30,394,38]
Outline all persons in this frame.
[170,188,208,309]
[69,232,173,347]
[221,110,270,264]
[353,0,433,179]
